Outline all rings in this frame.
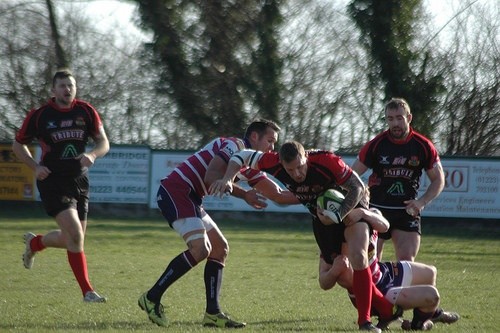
[406,207,414,214]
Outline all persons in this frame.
[138,118,302,328]
[207,141,406,333]
[350,96,446,322]
[12,69,107,303]
[317,183,459,333]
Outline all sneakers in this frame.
[22,232,36,268]
[138,293,170,328]
[202,312,246,328]
[83,290,107,304]
[359,310,460,333]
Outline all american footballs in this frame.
[317,189,345,214]
